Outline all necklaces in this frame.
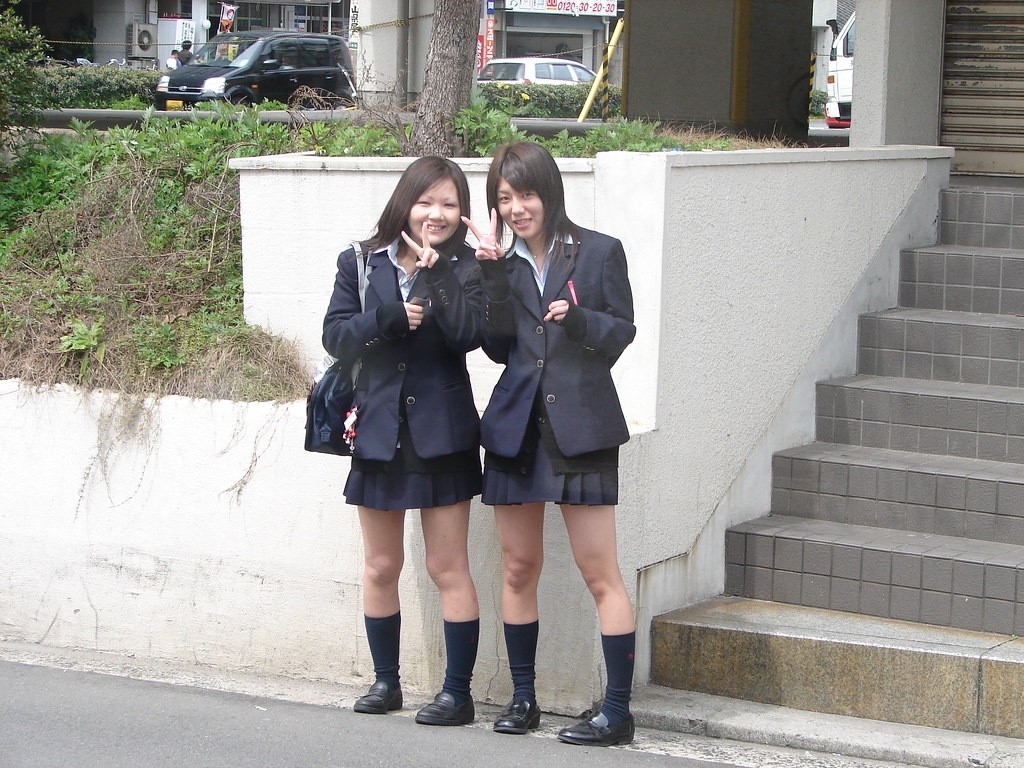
[405,250,416,262]
[531,254,536,259]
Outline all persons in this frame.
[166,50,182,71]
[323,156,483,726]
[178,40,193,66]
[461,143,636,746]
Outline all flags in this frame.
[214,6,239,62]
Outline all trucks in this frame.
[825,9,856,129]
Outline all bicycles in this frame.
[101,58,158,72]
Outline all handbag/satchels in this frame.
[304,240,374,455]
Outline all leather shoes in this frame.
[416,691,474,726]
[354,680,403,714]
[493,697,541,734]
[557,709,635,747]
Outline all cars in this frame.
[154,31,358,113]
[476,57,598,87]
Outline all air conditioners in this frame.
[126,23,157,59]
